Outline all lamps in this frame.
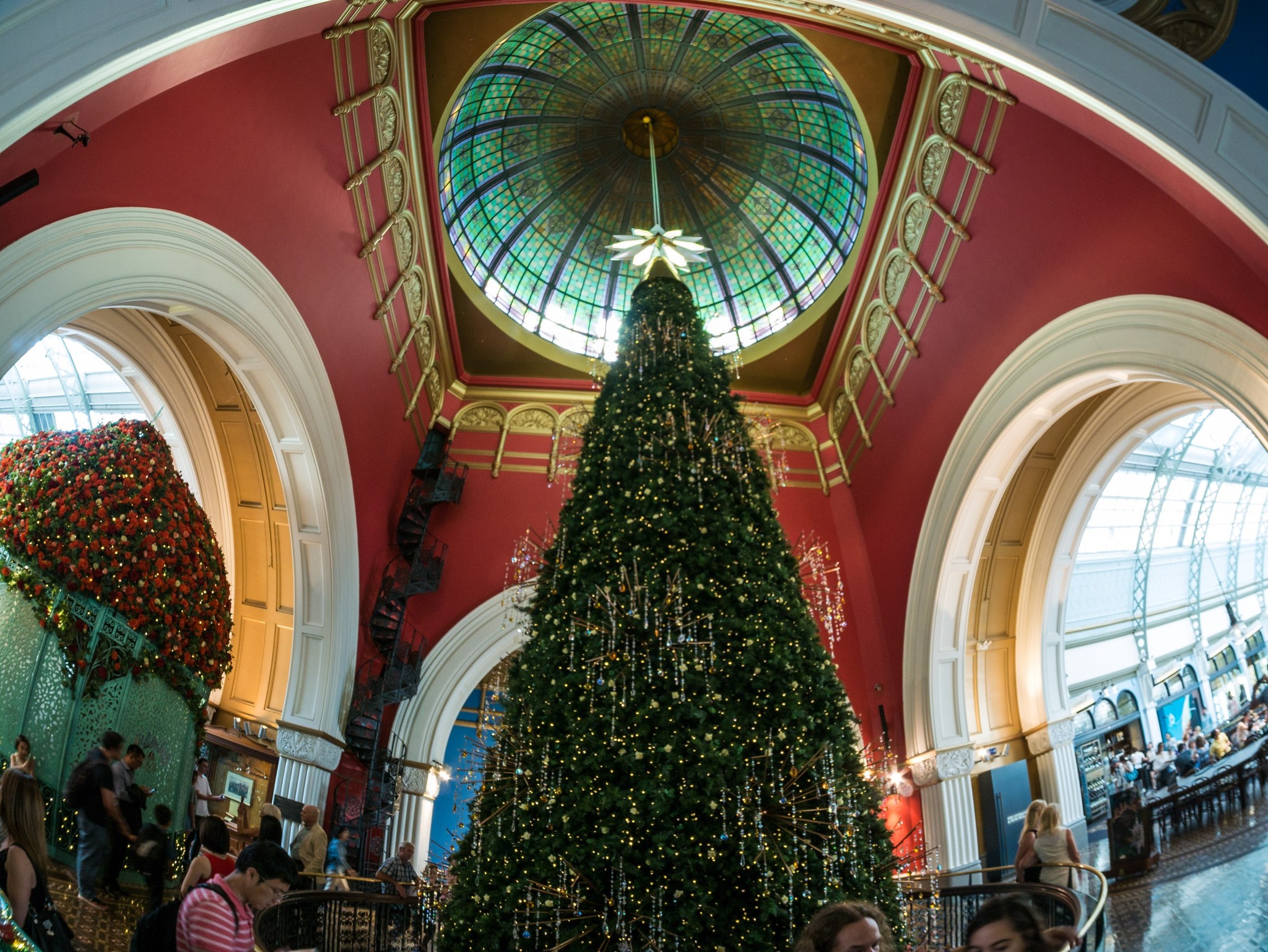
[258,725,268,739]
[243,722,252,735]
[233,717,239,730]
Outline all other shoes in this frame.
[102,884,123,900]
[77,894,108,910]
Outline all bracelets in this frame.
[237,816,244,821]
[1077,870,1083,873]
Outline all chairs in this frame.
[1146,734,1260,842]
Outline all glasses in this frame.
[257,873,286,901]
[132,755,142,766]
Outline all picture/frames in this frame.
[223,770,256,807]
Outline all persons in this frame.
[1107,674,1268,794]
[71,728,174,916]
[180,816,236,899]
[788,894,1083,952]
[0,767,76,952]
[374,841,420,952]
[410,852,460,952]
[10,734,36,777]
[236,794,282,846]
[175,840,298,952]
[290,804,328,891]
[184,757,225,838]
[320,823,358,912]
[1013,799,1084,891]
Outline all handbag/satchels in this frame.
[27,911,76,952]
[1123,768,1138,782]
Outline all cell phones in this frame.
[148,788,157,793]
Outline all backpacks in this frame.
[65,759,108,809]
[130,883,238,952]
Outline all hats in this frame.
[1112,758,1118,765]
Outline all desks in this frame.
[1142,736,1268,810]
